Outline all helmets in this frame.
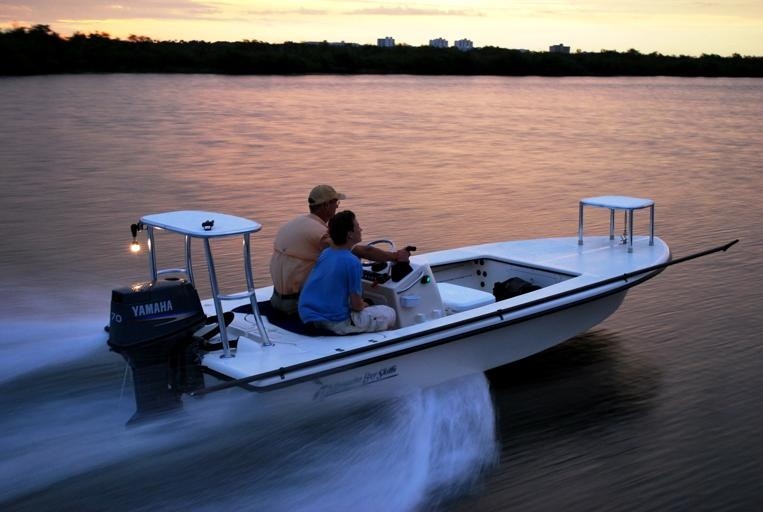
[308,185,346,206]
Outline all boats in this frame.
[101,194,674,430]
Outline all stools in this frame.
[437,279,495,313]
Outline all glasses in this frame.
[331,199,340,206]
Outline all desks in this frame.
[578,195,655,252]
[139,210,272,359]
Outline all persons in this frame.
[296,210,396,335]
[268,184,410,315]
